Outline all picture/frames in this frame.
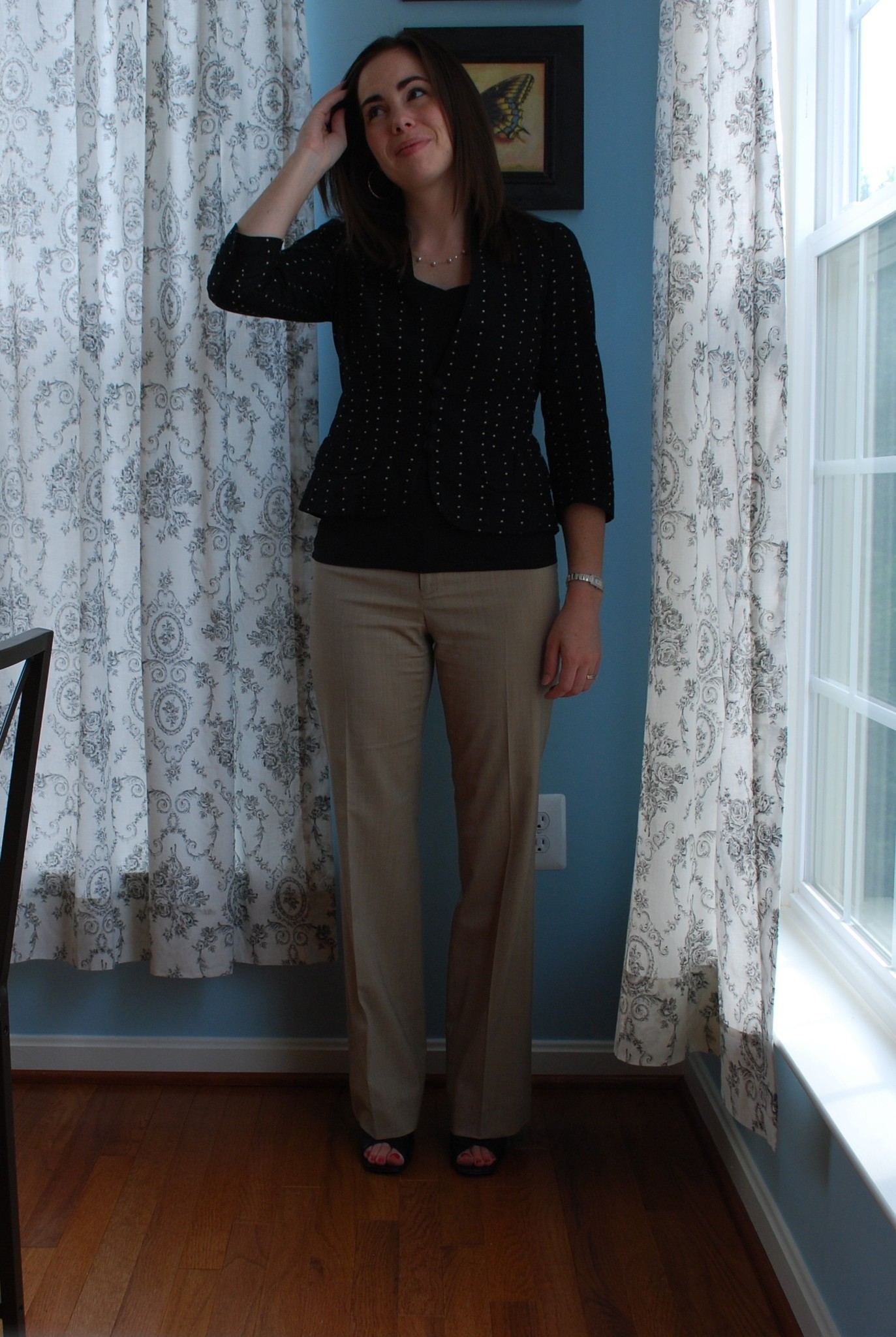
[401,25,585,210]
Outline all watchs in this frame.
[566,573,604,593]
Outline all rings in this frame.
[587,675,594,679]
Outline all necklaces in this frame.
[412,250,470,266]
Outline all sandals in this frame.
[449,1133,511,1174]
[358,1130,415,1175]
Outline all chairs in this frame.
[0,627,54,1337]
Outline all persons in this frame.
[206,32,617,1176]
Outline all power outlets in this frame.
[534,794,566,870]
